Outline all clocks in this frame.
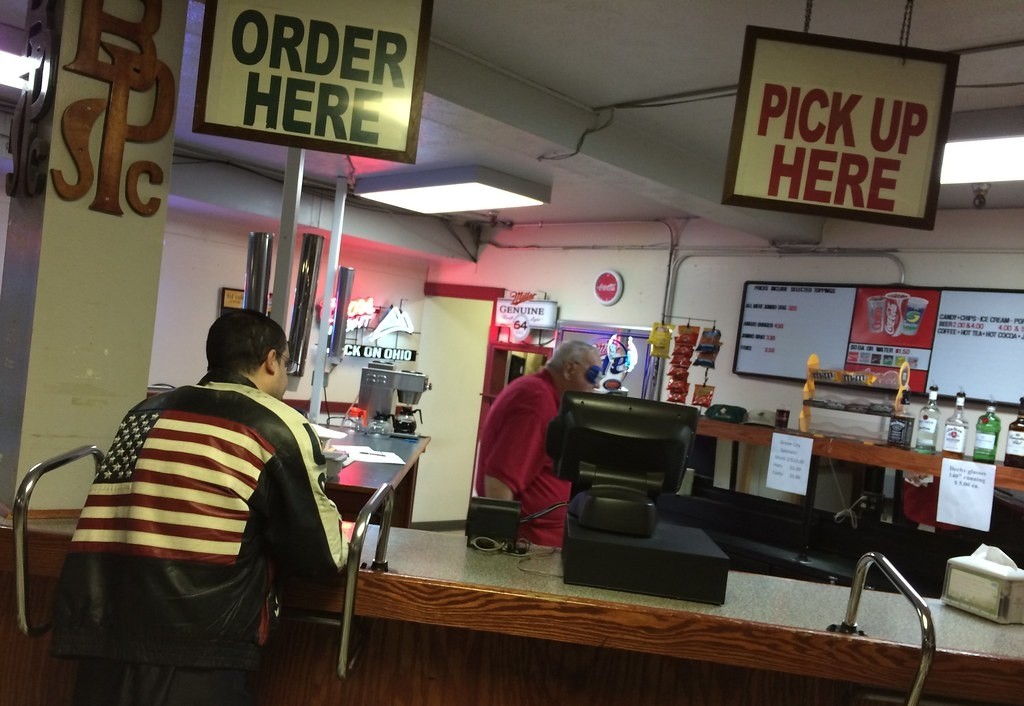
[593,271,623,307]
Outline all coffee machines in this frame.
[359,359,432,440]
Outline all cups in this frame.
[867,296,885,333]
[884,292,910,337]
[900,297,929,336]
[775,408,790,429]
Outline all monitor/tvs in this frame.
[552,392,699,494]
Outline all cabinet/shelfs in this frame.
[695,414,1024,598]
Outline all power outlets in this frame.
[859,491,878,512]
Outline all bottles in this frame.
[940,390,968,460]
[886,387,914,450]
[1004,397,1024,470]
[916,385,940,455]
[972,397,1001,465]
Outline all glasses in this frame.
[276,352,302,375]
[573,360,604,384]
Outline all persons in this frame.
[475,341,603,548]
[50,309,349,706]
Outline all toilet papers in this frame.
[675,468,695,496]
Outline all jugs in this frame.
[341,406,367,436]
[368,410,395,439]
[393,405,424,434]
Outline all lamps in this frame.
[353,164,552,215]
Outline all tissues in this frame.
[940,543,1024,625]
